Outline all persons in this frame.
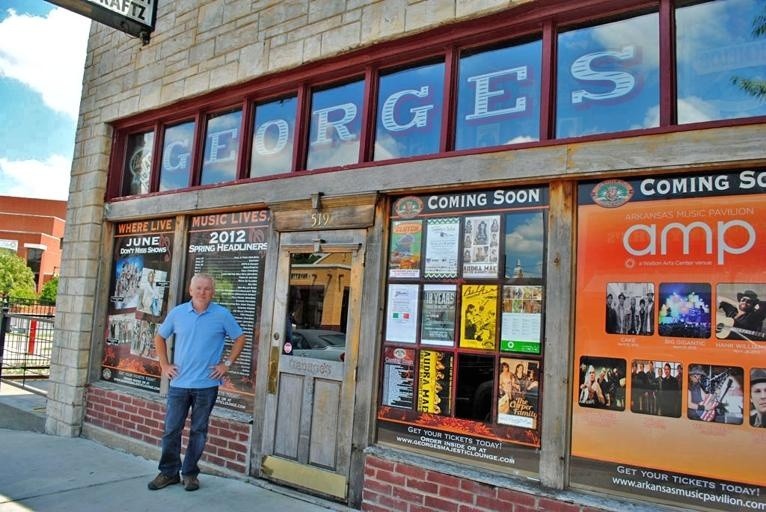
[499,360,538,411]
[465,302,496,340]
[399,371,409,378]
[578,285,765,430]
[147,273,246,492]
[433,351,445,416]
[464,219,498,264]
[110,262,163,359]
[282,287,301,355]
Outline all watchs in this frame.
[223,360,232,368]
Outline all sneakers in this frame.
[182,475,202,492]
[148,472,181,491]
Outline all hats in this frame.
[687,365,709,378]
[750,368,766,385]
[618,292,627,300]
[646,289,655,297]
[737,289,760,305]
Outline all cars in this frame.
[288,329,345,362]
